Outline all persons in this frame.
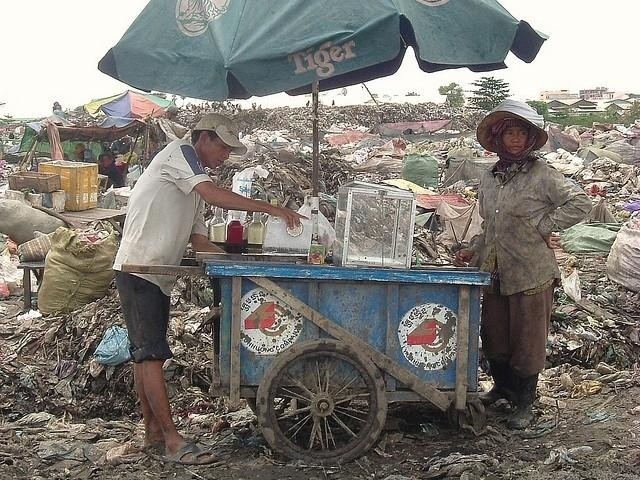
[176,100,241,116]
[251,101,263,112]
[453,101,593,429]
[113,113,308,466]
[62,138,132,186]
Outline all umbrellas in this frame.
[98,0,550,196]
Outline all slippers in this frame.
[151,443,217,464]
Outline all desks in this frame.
[17,257,46,312]
[58,205,126,238]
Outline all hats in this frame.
[477,99,548,154]
[193,114,247,156]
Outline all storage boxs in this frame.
[4,158,99,211]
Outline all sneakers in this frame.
[507,404,534,428]
[479,388,509,406]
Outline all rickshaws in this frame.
[120,251,491,467]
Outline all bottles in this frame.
[227,209,243,250]
[209,206,227,244]
[247,211,265,248]
